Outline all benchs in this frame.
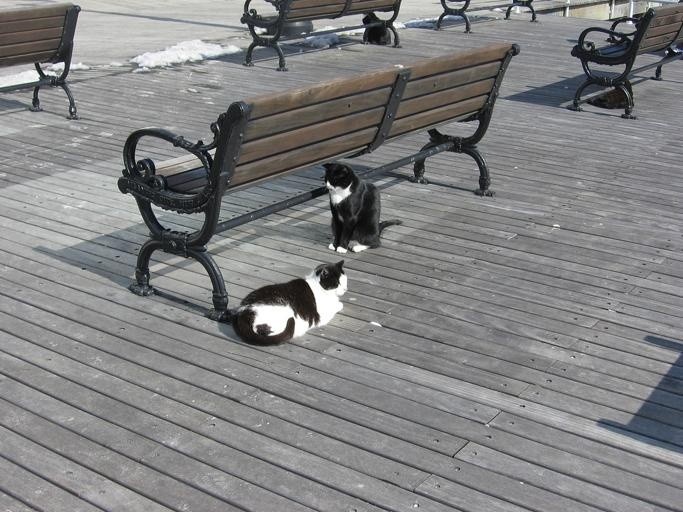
[1,3,83,123]
[433,3,537,37]
[236,1,403,73]
[116,39,524,318]
[569,0,683,120]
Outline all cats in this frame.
[321,162,404,254]
[231,259,348,347]
